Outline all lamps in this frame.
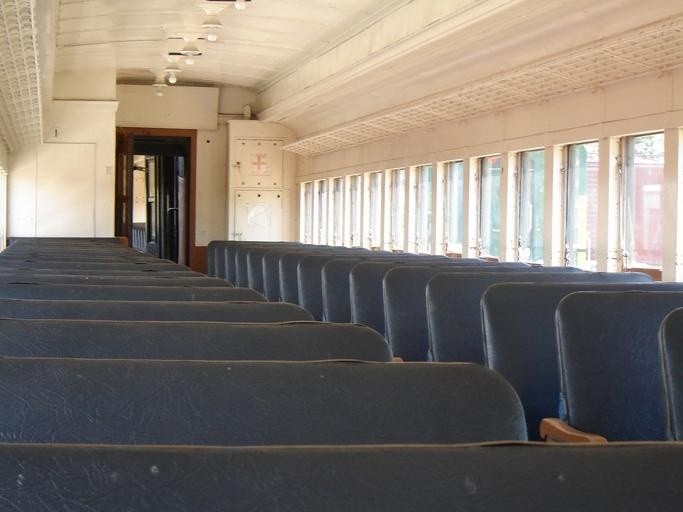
[152,0,249,97]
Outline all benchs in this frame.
[0,238,683,512]
